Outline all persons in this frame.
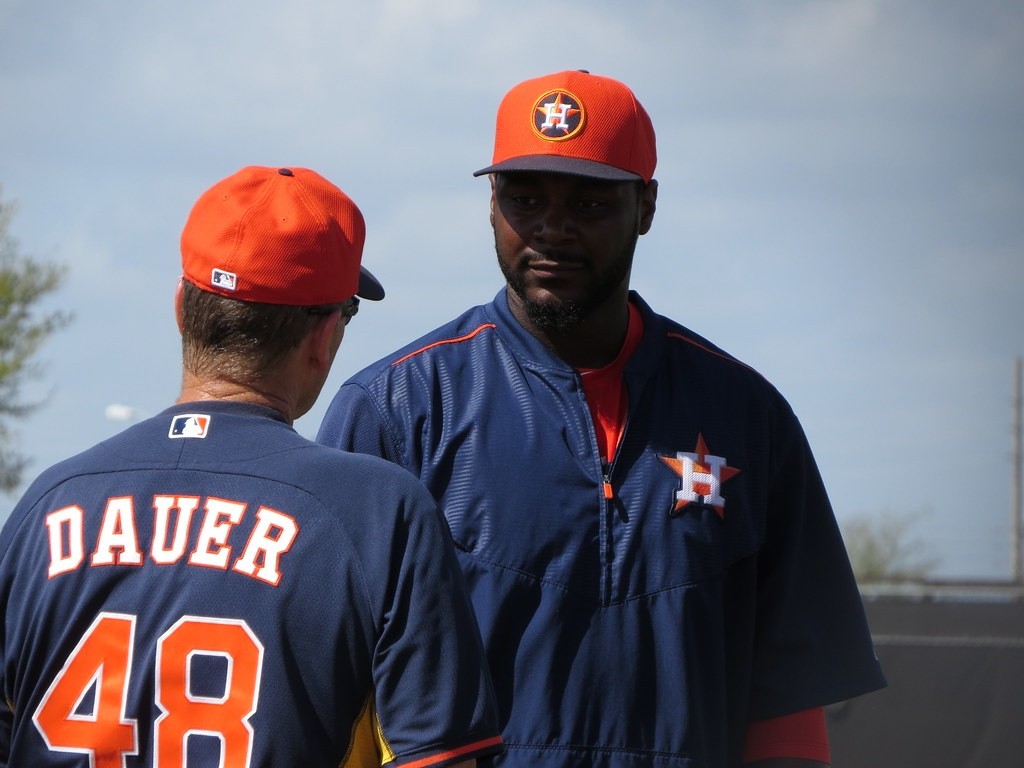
[307,64,894,768]
[1,162,514,766]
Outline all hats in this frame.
[472,69,657,180]
[180,166,386,308]
